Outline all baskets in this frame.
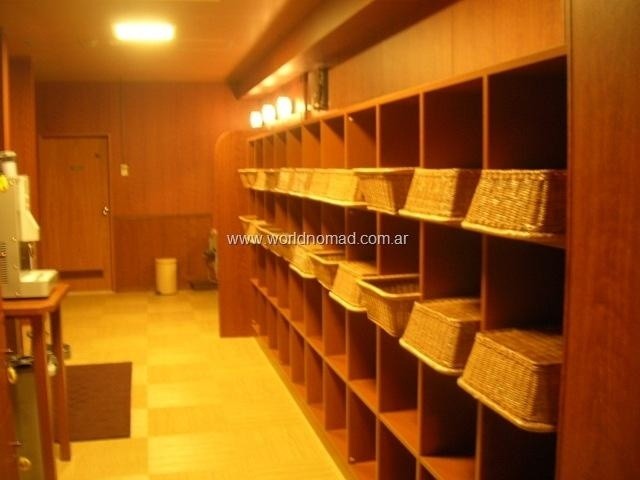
[455,326,563,434]
[328,258,376,313]
[352,166,415,217]
[355,273,419,338]
[398,166,481,223]
[237,167,367,208]
[397,296,480,378]
[237,214,345,292]
[460,168,567,244]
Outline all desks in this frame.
[1,284,72,480]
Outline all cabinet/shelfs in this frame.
[242,42,568,480]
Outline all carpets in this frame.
[40,360,133,443]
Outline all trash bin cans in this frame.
[155,258,177,295]
[10,353,51,480]
